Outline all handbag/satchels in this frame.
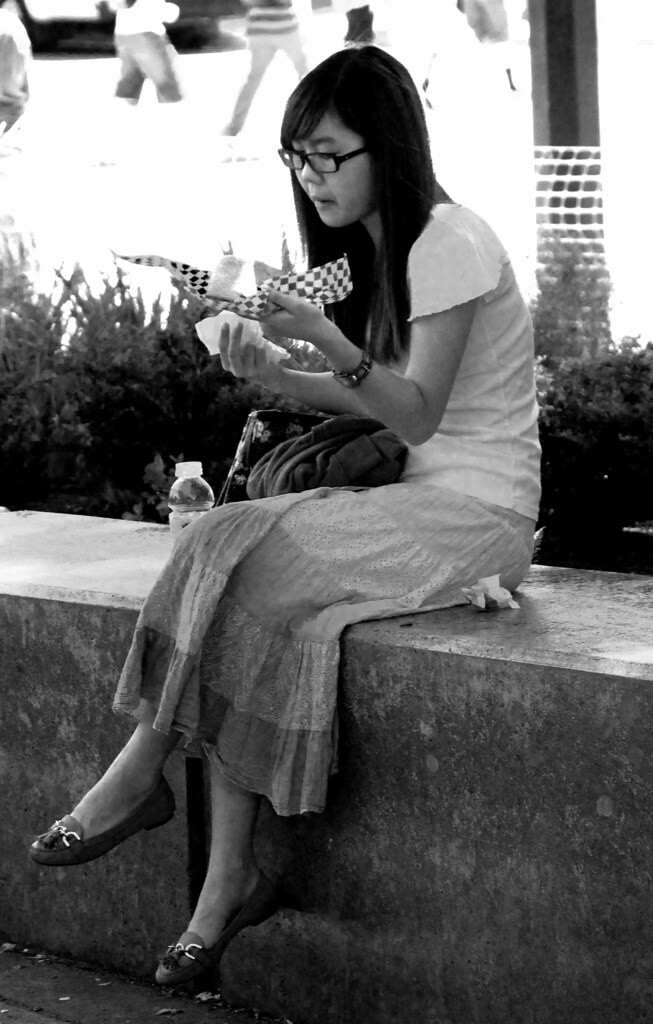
[217,410,330,506]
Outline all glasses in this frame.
[278,147,370,173]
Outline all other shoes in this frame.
[30,776,175,866]
[156,868,279,983]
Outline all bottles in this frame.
[167,462,214,548]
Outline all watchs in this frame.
[331,350,373,388]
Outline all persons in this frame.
[24,46,544,985]
